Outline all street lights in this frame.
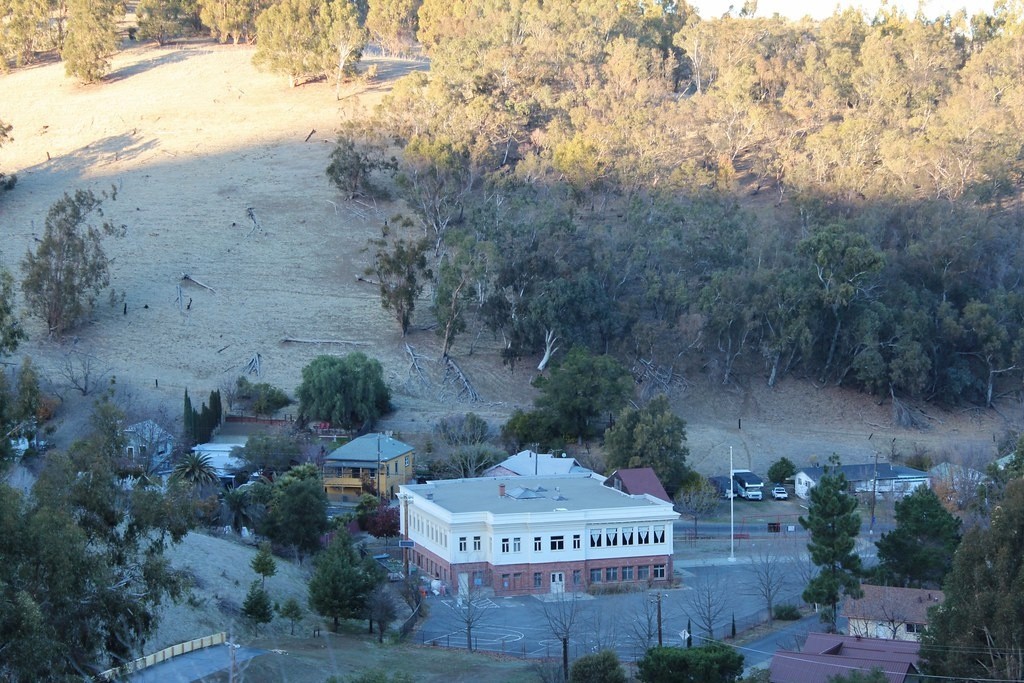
[648,592,668,649]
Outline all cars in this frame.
[771,487,789,500]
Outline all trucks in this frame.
[732,469,764,501]
